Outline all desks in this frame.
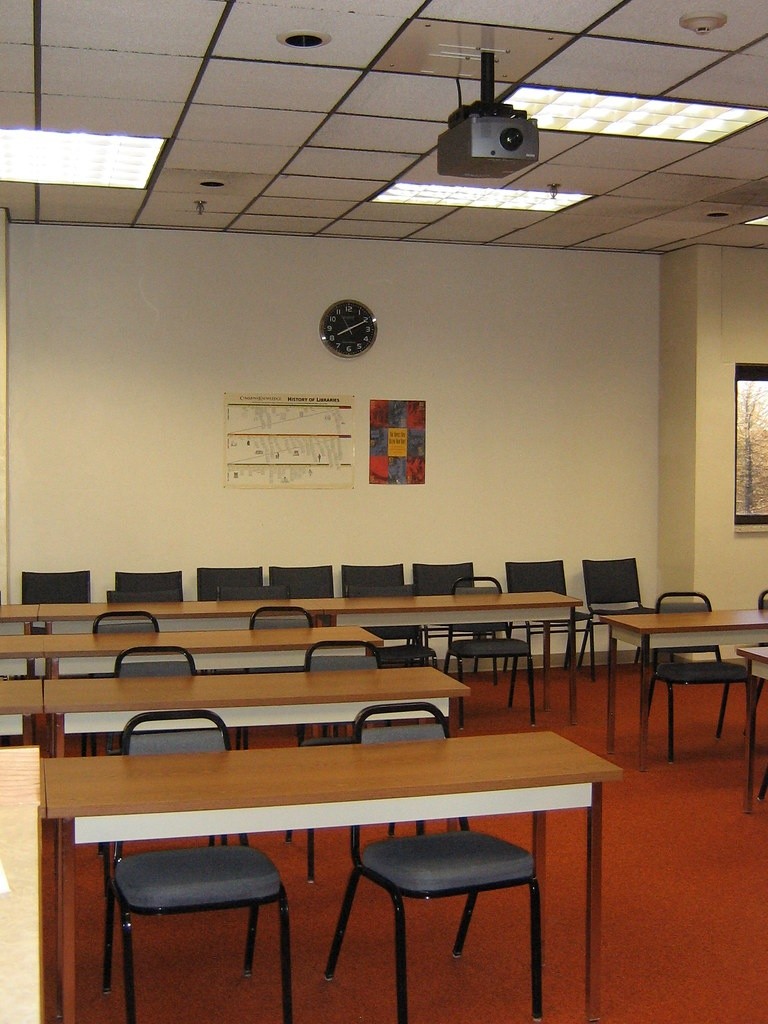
[311,592,583,725]
[37,599,324,635]
[44,731,623,1024]
[44,625,384,760]
[599,610,768,772]
[736,646,768,813]
[0,679,43,746]
[0,634,44,681]
[0,604,39,635]
[43,666,471,832]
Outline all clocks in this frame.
[319,300,378,359]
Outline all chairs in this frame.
[248,606,314,631]
[345,584,437,670]
[502,560,597,681]
[115,571,183,602]
[324,702,543,1024]
[92,611,159,631]
[98,646,228,860]
[444,577,535,729]
[743,589,768,737]
[106,590,182,602]
[196,565,334,601]
[21,570,91,604]
[646,591,749,765]
[100,709,293,1024]
[413,562,476,596]
[285,640,384,883]
[577,558,658,682]
[342,564,404,594]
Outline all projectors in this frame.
[438,114,540,178]
